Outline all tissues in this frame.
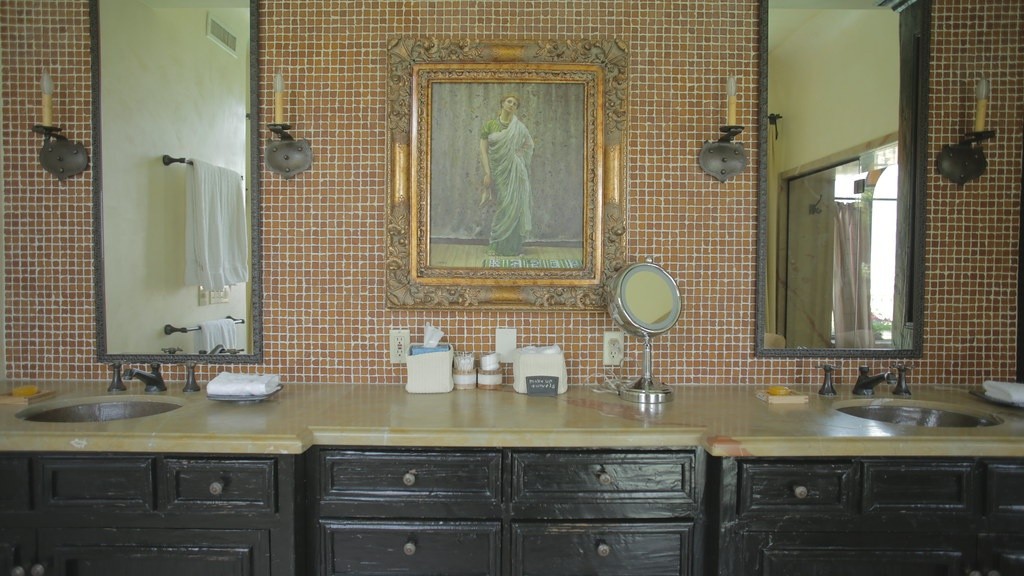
[405,321,454,393]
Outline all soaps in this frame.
[12,385,39,396]
[766,383,792,396]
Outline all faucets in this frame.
[161,345,183,355]
[199,344,244,355]
[851,365,898,397]
[123,363,172,392]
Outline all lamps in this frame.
[32,67,87,181]
[698,72,746,183]
[936,73,995,187]
[264,69,312,181]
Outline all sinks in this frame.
[831,398,1004,428]
[13,396,183,423]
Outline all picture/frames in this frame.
[383,37,630,314]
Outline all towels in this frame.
[194,319,236,355]
[205,371,281,395]
[983,379,1023,404]
[185,157,249,293]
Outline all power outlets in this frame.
[602,330,624,366]
[389,330,410,364]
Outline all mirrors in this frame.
[753,0,932,361]
[86,0,264,366]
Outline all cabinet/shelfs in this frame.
[0,444,1024,576]
